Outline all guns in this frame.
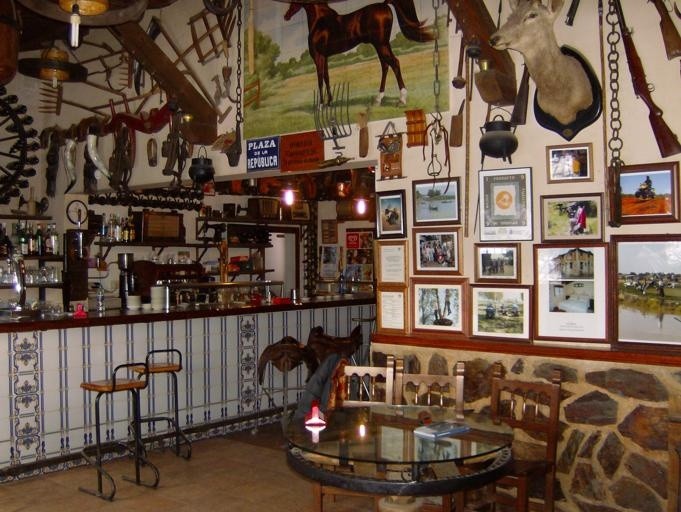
[511,61,529,125]
[613,0,681,158]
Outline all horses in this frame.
[284,1,439,107]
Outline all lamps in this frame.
[69,1,82,48]
[17,36,89,82]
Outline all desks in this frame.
[284,406,514,512]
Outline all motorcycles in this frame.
[385,211,397,225]
[486,305,496,320]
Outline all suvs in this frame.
[623,274,641,290]
[634,182,656,200]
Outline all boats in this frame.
[421,200,426,205]
[441,200,453,204]
[430,204,439,210]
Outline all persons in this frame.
[375,139,681,347]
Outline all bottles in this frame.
[0,255,64,286]
[340,272,346,294]
[143,252,193,275]
[0,221,61,255]
[102,211,136,242]
[96,285,106,312]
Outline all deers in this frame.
[488,1,593,125]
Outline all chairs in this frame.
[455,362,561,511]
[127,349,192,468]
[392,360,467,511]
[312,355,395,511]
[77,362,160,502]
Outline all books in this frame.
[413,418,470,439]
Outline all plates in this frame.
[126,286,168,310]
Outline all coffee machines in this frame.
[118,252,137,305]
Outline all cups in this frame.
[7,298,63,315]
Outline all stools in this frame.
[254,335,319,438]
[305,325,369,401]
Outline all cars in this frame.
[568,201,597,219]
